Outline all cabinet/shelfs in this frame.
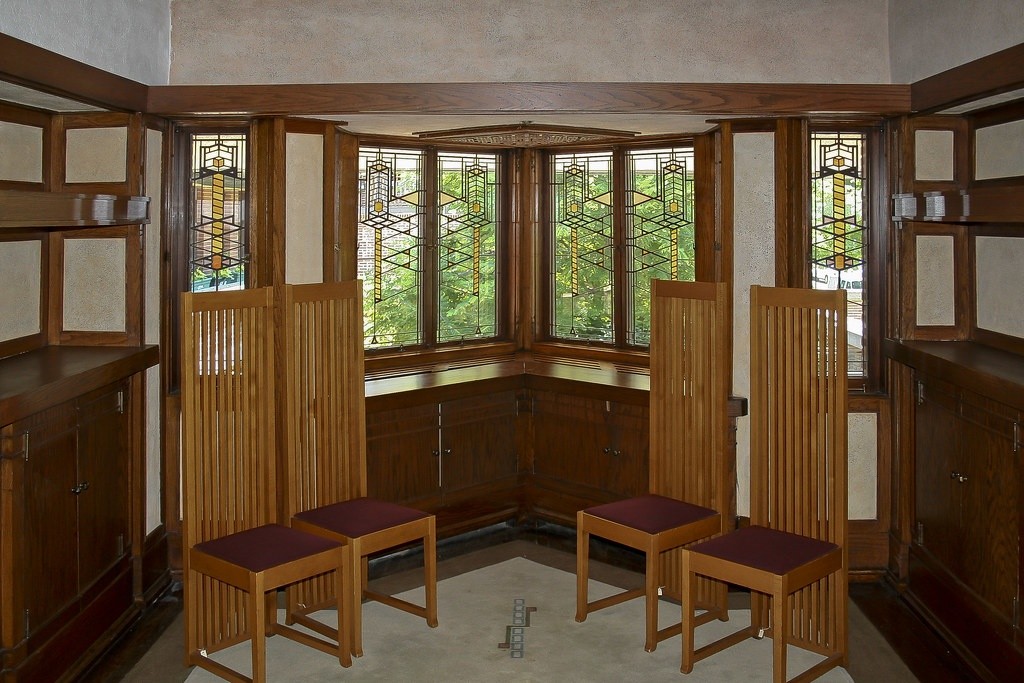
[890,40,1024,683]
[366,388,521,538]
[0,99,164,683]
[522,390,649,524]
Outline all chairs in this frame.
[573,278,730,648]
[678,285,847,683]
[279,280,440,659]
[168,285,354,683]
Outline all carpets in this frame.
[185,554,855,683]
